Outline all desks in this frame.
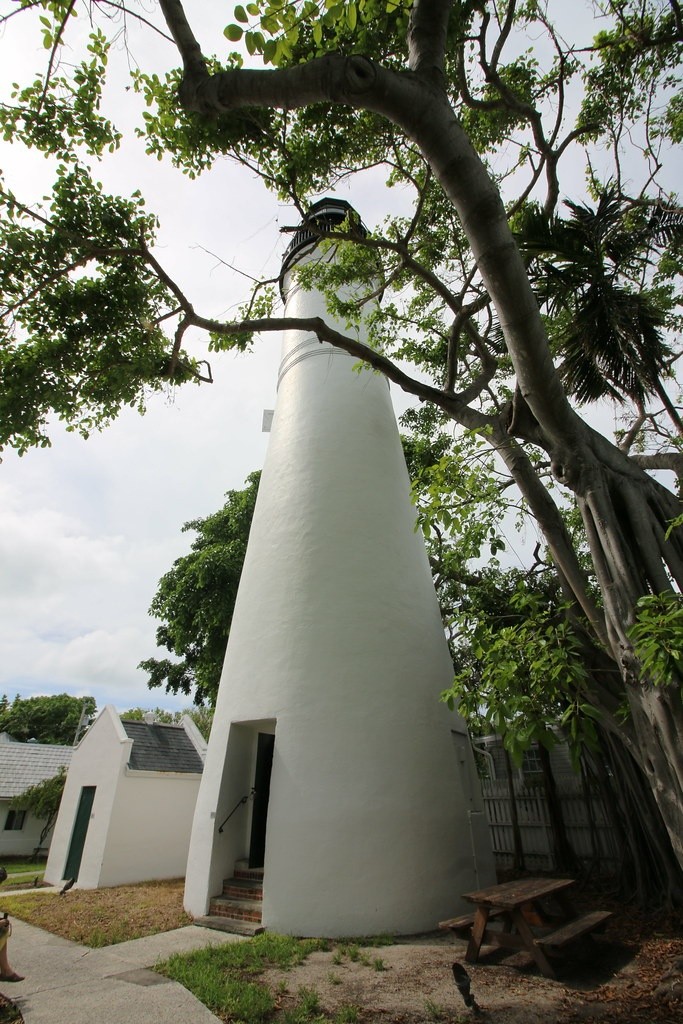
[460,876,598,981]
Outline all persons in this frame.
[0,865,25,983]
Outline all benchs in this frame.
[438,907,514,964]
[537,911,615,982]
